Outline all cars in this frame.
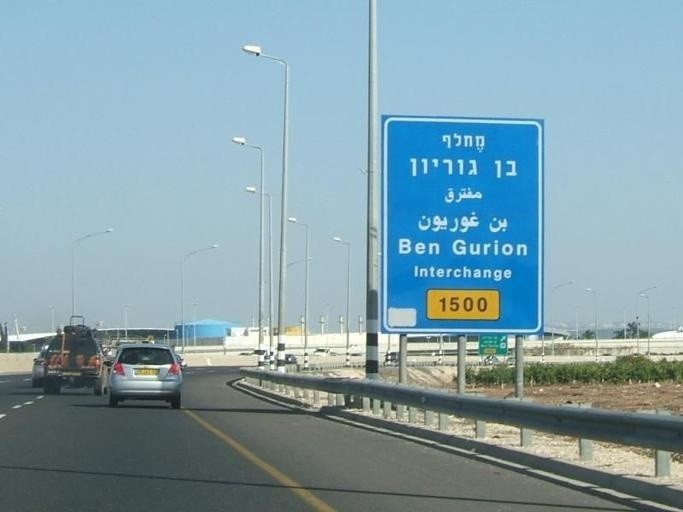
[485,356,500,365]
[32,316,186,408]
[285,354,296,364]
[314,349,330,356]
[506,357,516,365]
[390,352,399,361]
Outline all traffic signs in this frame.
[479,335,507,356]
[380,113,541,334]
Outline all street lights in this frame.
[288,216,310,369]
[232,44,290,372]
[70,228,113,324]
[180,244,219,349]
[333,237,351,369]
[549,279,660,363]
